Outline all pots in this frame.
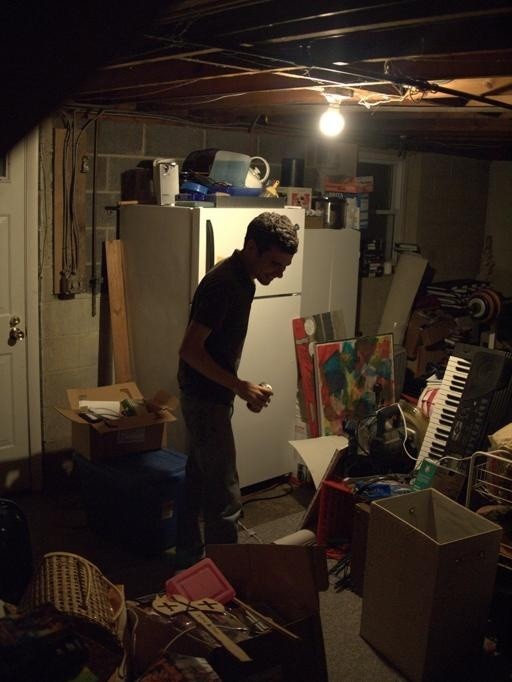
[313,197,345,230]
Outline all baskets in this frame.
[21,551,124,679]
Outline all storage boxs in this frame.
[128,539,334,682]
[354,485,506,680]
[51,376,192,561]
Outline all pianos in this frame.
[414,344,511,471]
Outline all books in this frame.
[120,399,152,416]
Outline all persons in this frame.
[176,214,299,572]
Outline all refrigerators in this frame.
[115,201,307,490]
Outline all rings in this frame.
[260,393,267,399]
